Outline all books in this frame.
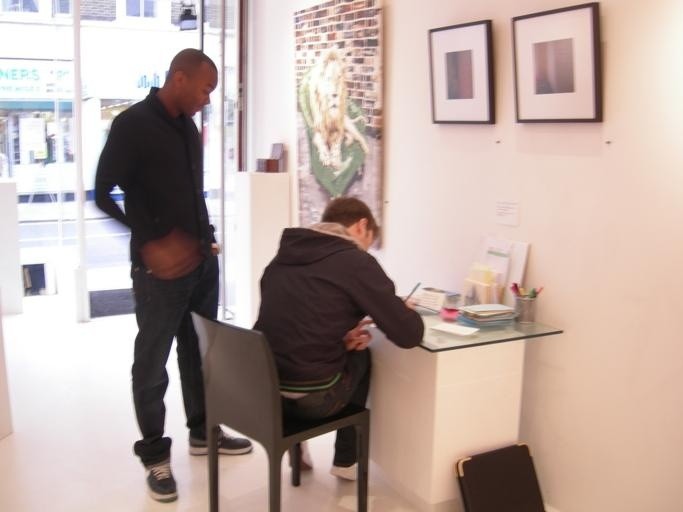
[412,245,520,327]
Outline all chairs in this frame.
[457,441,546,511]
[189,310,371,512]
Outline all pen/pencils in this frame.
[404,282,421,302]
[511,282,544,322]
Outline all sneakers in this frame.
[289,441,312,469]
[190,430,252,455]
[330,462,358,481]
[145,461,178,501]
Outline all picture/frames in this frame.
[511,1,601,123]
[428,19,497,124]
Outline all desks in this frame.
[363,316,563,511]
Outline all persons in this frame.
[92,47,251,502]
[253,196,423,483]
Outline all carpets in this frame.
[89,288,136,319]
